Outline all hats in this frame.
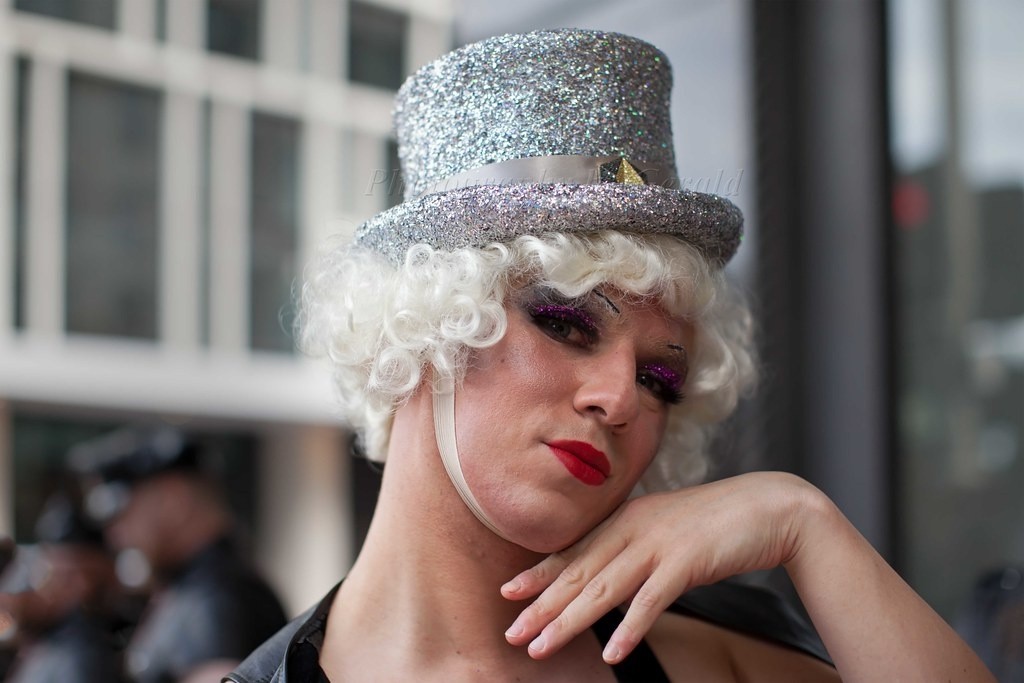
[358,28,744,264]
[41,504,111,549]
[70,428,217,500]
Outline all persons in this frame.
[0,424,290,683]
[219,28,1001,683]
